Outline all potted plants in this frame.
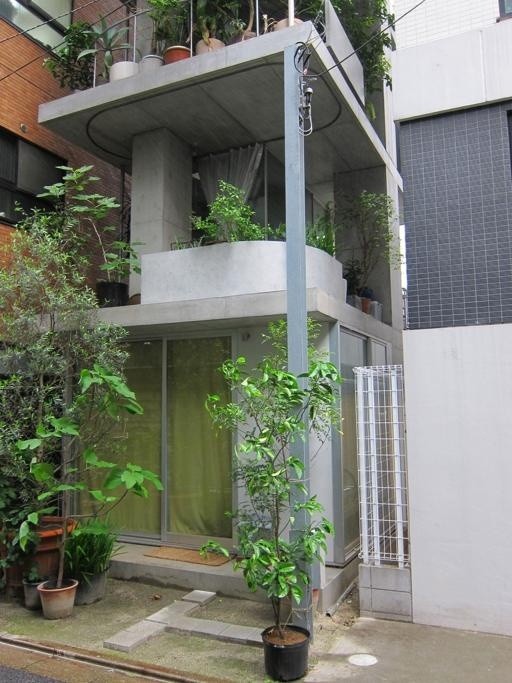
[74,0,228,80]
[334,188,404,320]
[209,315,343,683]
